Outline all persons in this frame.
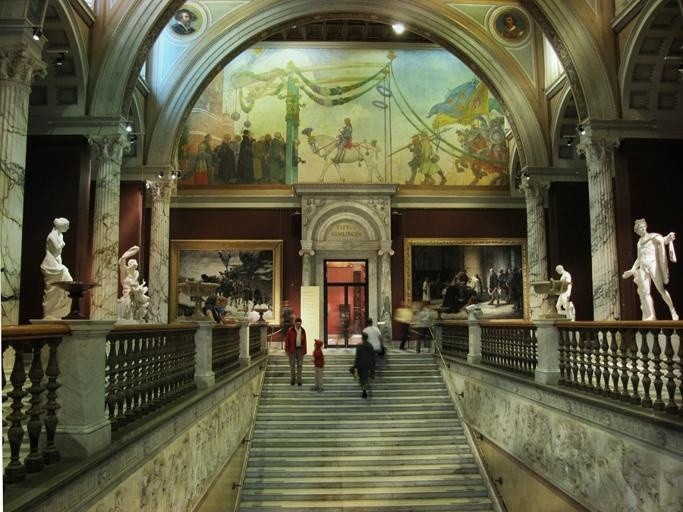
[202,294,225,325]
[216,296,236,323]
[170,8,199,33]
[416,302,435,353]
[330,117,352,164]
[283,317,307,386]
[499,11,524,39]
[361,318,385,378]
[178,127,308,188]
[352,332,377,398]
[117,244,151,320]
[310,338,326,391]
[417,129,448,184]
[38,216,75,321]
[201,264,264,312]
[462,296,484,320]
[421,263,522,312]
[393,298,413,351]
[622,217,682,321]
[405,133,436,186]
[555,263,576,320]
[468,113,511,175]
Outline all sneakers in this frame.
[290,382,302,387]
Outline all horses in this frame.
[306,134,384,184]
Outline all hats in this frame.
[313,338,323,347]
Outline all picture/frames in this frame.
[167,239,283,327]
[402,237,530,324]
